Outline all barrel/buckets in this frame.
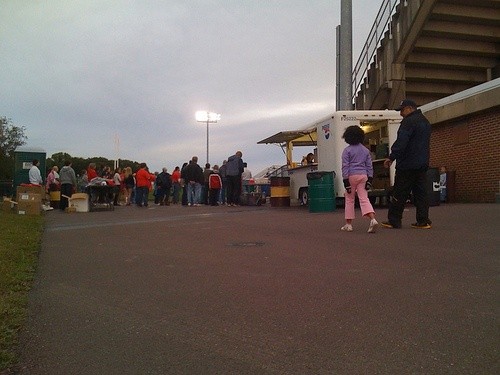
[306,171,336,213]
[269,176,290,208]
[426,167,441,206]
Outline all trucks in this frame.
[255,109,404,209]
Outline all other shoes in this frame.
[381,221,394,228]
[410,219,432,229]
[340,223,353,232]
[368,218,378,233]
[188,202,201,207]
[227,202,238,207]
[43,206,54,211]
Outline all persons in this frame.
[45,161,76,210]
[378,99,433,229]
[436,166,449,203]
[28,159,43,187]
[154,152,252,206]
[337,125,378,232]
[301,152,314,164]
[80,162,152,206]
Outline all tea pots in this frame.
[260,184,270,203]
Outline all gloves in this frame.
[343,179,351,193]
[365,177,373,190]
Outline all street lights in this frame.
[196,110,221,164]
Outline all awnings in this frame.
[257,127,317,154]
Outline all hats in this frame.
[395,98,416,111]
